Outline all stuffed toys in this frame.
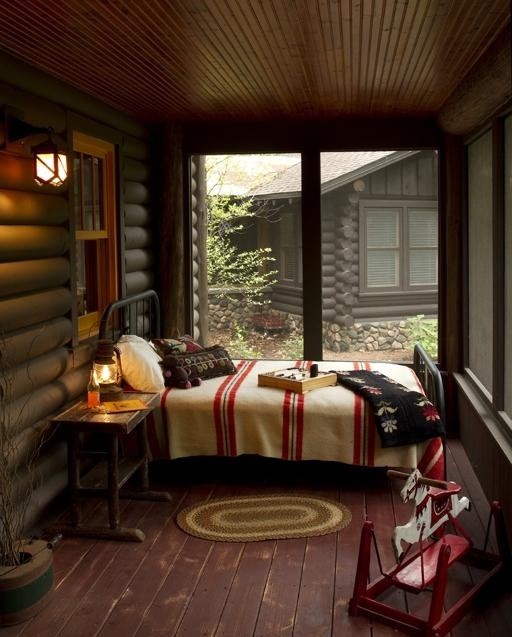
[157,351,201,390]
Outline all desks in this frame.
[42,390,172,542]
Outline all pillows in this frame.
[114,334,166,392]
[152,334,204,358]
[171,344,237,380]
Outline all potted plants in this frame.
[1,324,53,626]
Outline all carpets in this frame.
[175,493,354,543]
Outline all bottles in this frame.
[87,369,100,409]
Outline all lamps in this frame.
[94,339,123,400]
[8,116,69,190]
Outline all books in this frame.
[99,398,151,413]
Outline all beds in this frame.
[99,289,447,485]
